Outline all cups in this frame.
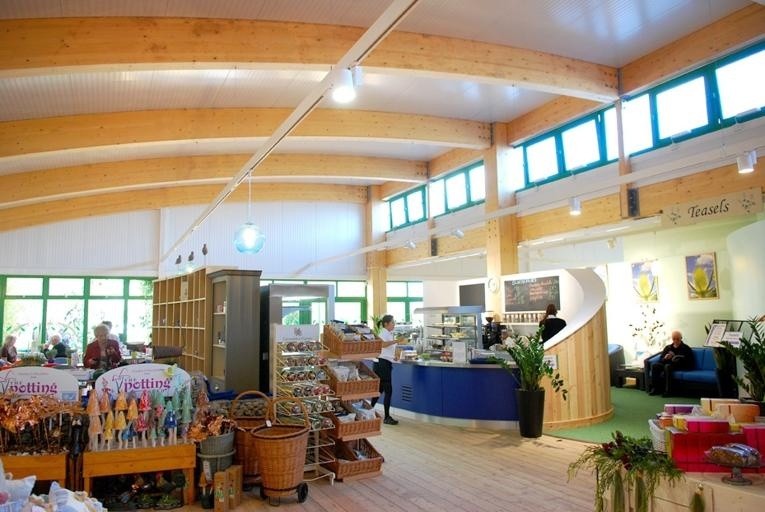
[70,353,78,368]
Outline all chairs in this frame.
[0,344,76,368]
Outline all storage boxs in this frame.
[649,418,765,474]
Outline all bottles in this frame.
[201,460,213,508]
[282,415,333,429]
[281,370,326,381]
[282,341,320,351]
[285,356,327,366]
[282,384,331,396]
[282,399,332,413]
[503,312,543,322]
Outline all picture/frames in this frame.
[684,253,719,300]
[632,261,658,300]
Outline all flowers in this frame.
[571,431,682,493]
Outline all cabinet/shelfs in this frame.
[84,364,197,508]
[595,465,764,512]
[2,367,79,495]
[206,270,260,393]
[415,307,481,349]
[270,326,334,491]
[151,266,208,392]
[322,325,391,479]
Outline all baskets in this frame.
[231,324,384,498]
[648,419,668,455]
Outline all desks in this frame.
[613,366,645,390]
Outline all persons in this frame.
[368,314,403,425]
[0,319,152,378]
[648,330,697,400]
[537,304,567,342]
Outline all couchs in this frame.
[644,345,737,398]
[607,342,626,383]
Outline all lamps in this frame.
[405,240,415,250]
[175,228,210,263]
[653,231,662,277]
[453,229,464,238]
[533,249,544,258]
[233,174,264,256]
[605,236,615,250]
[568,196,582,216]
[733,151,758,174]
[331,62,362,105]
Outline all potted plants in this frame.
[494,329,567,438]
[730,321,765,415]
